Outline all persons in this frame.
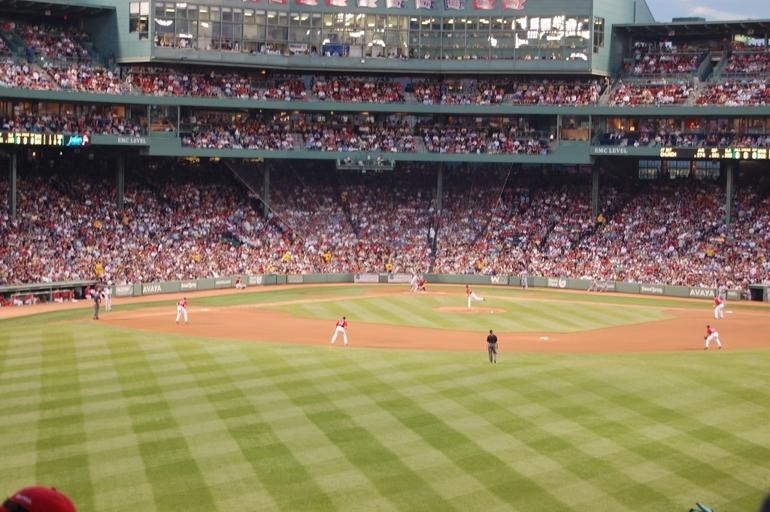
[0,16,769,313]
[176,297,187,324]
[704,325,721,349]
[487,330,497,363]
[93,289,101,320]
[713,295,724,319]
[330,316,348,345]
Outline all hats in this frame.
[0,487,78,510]
